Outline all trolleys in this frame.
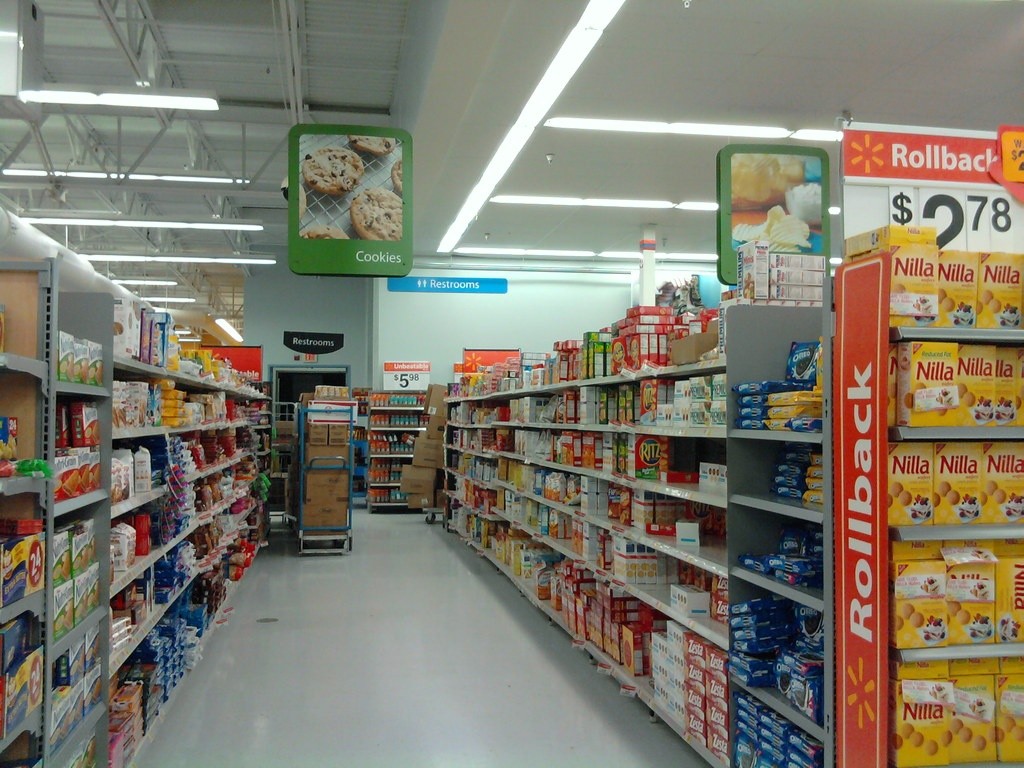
[288,405,353,555]
[422,507,443,523]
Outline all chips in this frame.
[730,205,811,253]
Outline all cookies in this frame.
[298,134,403,241]
[779,667,793,693]
[795,347,815,377]
[803,609,822,637]
[739,741,754,768]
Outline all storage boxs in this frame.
[419,431,427,438]
[412,437,444,469]
[307,443,350,465]
[435,490,444,508]
[400,479,433,493]
[425,383,447,418]
[329,424,347,446]
[426,415,443,439]
[310,424,328,446]
[408,494,434,508]
[302,471,349,535]
[403,465,437,481]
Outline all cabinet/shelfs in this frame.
[367,389,430,514]
[351,388,371,508]
[443,252,1024,768]
[0,205,275,768]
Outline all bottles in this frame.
[370,394,431,502]
